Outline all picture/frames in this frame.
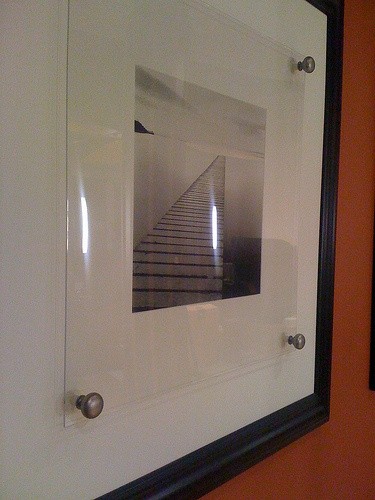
[0,0,345,500]
